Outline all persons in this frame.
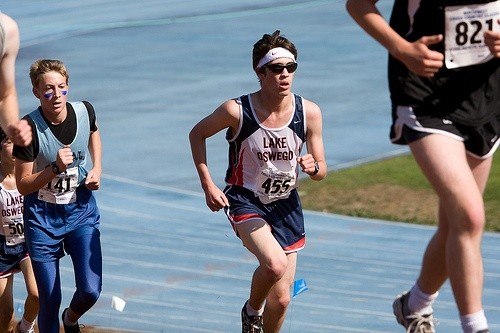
[0,11,102,333]
[189,30,328,333]
[345,0,500,333]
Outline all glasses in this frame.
[264,63,297,73]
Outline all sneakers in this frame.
[15,320,35,333]
[62,308,81,333]
[393,290,435,333]
[242,299,263,333]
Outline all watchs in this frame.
[52,161,62,175]
[307,161,320,176]
